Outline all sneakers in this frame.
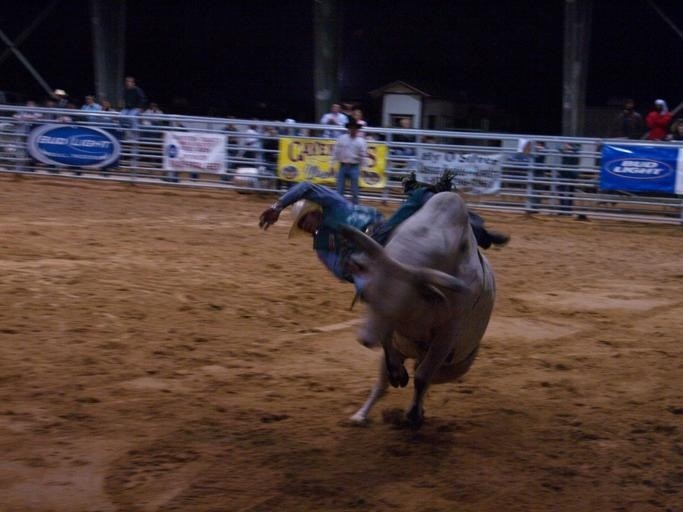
[477,233,508,249]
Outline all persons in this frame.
[521,141,549,213]
[11,76,166,177]
[392,115,416,156]
[256,183,513,305]
[211,101,374,208]
[608,94,683,141]
[555,142,582,217]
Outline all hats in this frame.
[345,119,361,129]
[289,198,323,238]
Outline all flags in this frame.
[160,130,228,177]
[277,136,389,191]
[597,141,682,197]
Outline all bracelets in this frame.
[270,199,283,213]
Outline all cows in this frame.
[333,189,496,429]
